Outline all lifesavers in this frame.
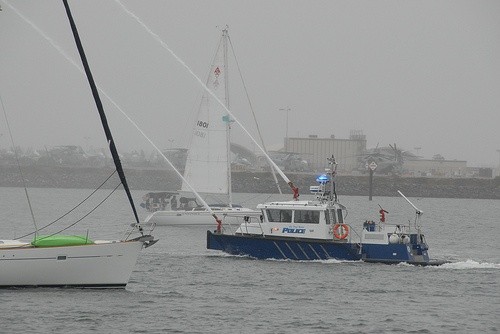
[333,223,349,240]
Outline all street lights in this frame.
[279,106,292,159]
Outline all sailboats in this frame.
[136,28,300,229]
[0,4,161,288]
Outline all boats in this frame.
[213,155,433,266]
